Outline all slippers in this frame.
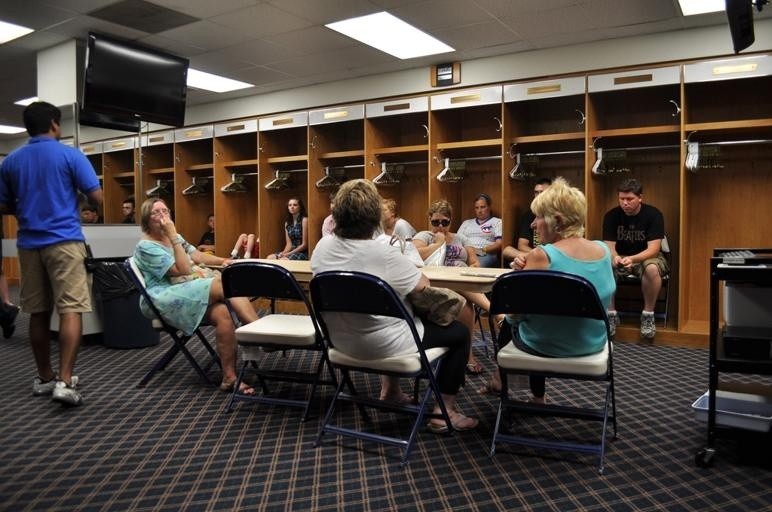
[427,413,479,433]
[480,381,513,396]
[466,362,483,375]
[220,379,256,396]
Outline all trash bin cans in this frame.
[85,255,161,348]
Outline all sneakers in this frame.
[52,375,84,406]
[640,314,656,339]
[607,313,620,338]
[32,375,59,395]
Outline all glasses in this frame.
[431,219,450,226]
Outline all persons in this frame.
[455,192,503,270]
[121,196,135,224]
[0,269,21,311]
[0,101,104,408]
[196,213,215,256]
[499,175,615,404]
[321,189,337,241]
[369,198,418,250]
[309,179,480,434]
[229,231,259,259]
[135,196,262,396]
[168,236,182,246]
[80,202,103,225]
[600,176,665,342]
[502,177,552,270]
[265,196,310,261]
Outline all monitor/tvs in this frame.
[726,0,755,55]
[80,28,190,132]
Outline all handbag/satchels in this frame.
[375,233,424,268]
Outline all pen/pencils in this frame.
[428,232,437,238]
